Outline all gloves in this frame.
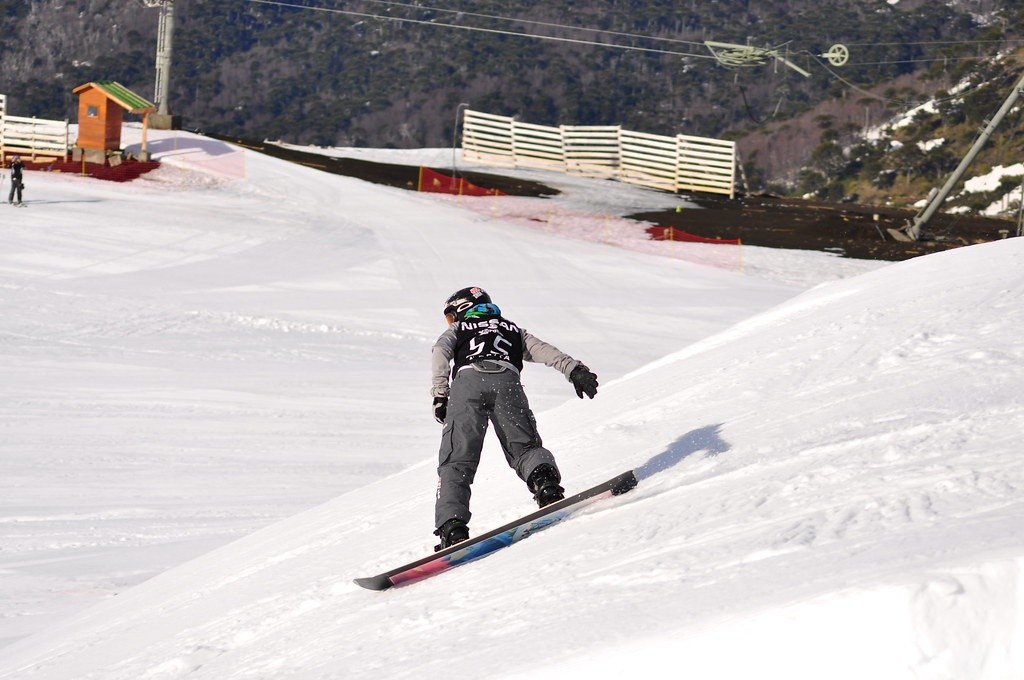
[571,365,598,399]
[433,397,448,424]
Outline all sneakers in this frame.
[434,518,469,551]
[527,463,565,509]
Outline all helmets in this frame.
[444,286,491,322]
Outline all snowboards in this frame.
[353,470,640,591]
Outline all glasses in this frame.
[445,313,455,325]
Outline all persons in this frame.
[430,287,598,551]
[9,155,24,206]
